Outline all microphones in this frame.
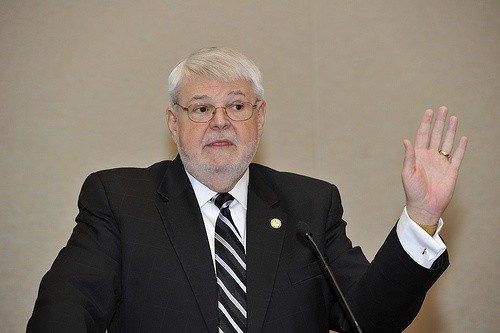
[296,221,362,333]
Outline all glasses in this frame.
[174,100,257,123]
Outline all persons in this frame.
[27,47,468,332]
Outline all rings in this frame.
[438,150,450,159]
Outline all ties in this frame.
[210,191,247,332]
